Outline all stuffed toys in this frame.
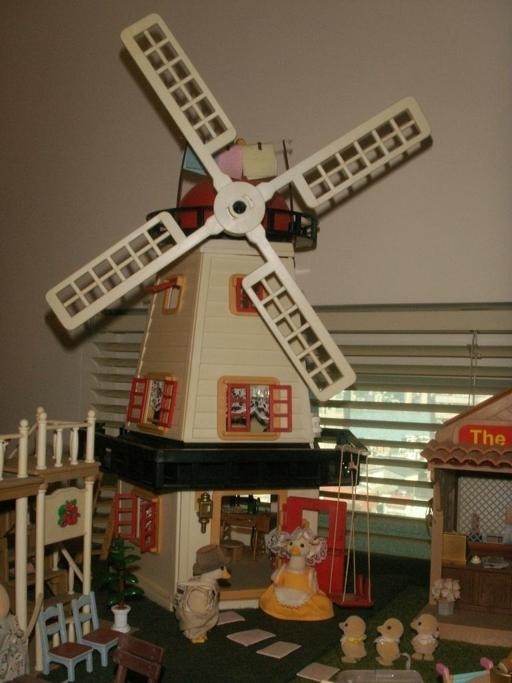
[372,616,403,667]
[408,612,441,664]
[175,542,232,645]
[335,615,368,665]
[258,519,335,622]
